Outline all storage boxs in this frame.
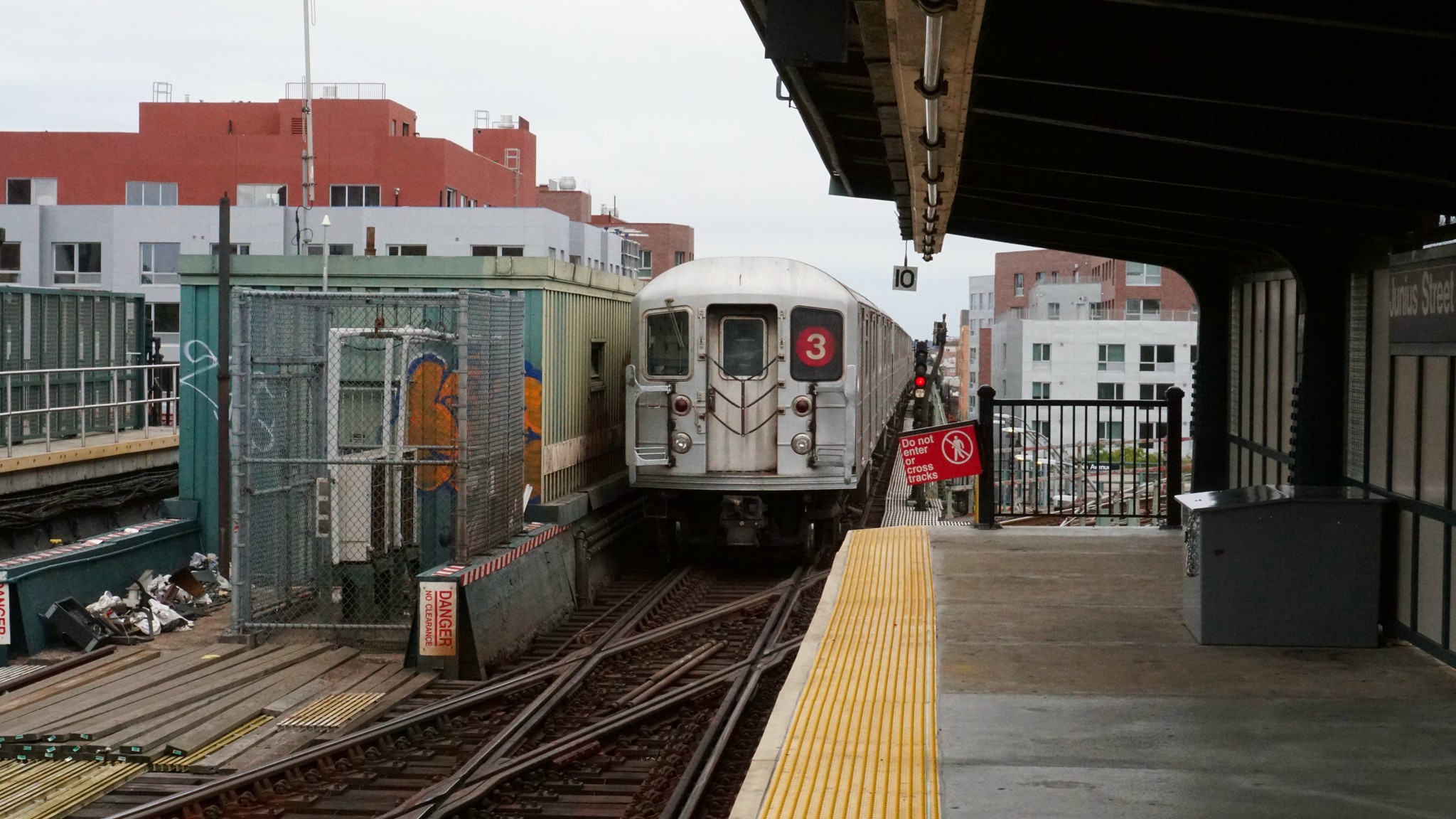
[44,595,115,652]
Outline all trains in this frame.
[623,254,914,564]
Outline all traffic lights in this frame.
[914,341,928,389]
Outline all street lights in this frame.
[993,414,1104,502]
